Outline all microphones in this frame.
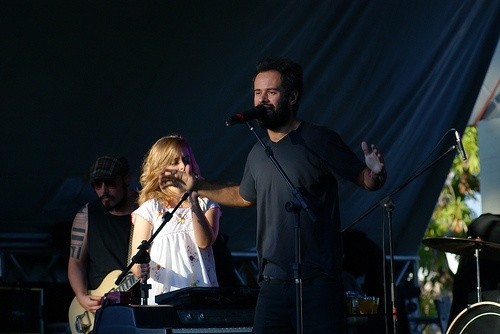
[455,130,466,160]
[225,104,268,127]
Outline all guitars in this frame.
[69,270,140,334]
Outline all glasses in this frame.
[92,178,118,188]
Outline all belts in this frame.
[264,275,281,280]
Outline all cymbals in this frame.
[423,237,500,258]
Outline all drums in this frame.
[444,301,500,334]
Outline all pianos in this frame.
[93,304,254,334]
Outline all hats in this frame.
[86,153,136,178]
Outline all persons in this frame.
[68,156,139,313]
[130,136,221,306]
[158,59,388,334]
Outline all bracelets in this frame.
[189,200,201,212]
